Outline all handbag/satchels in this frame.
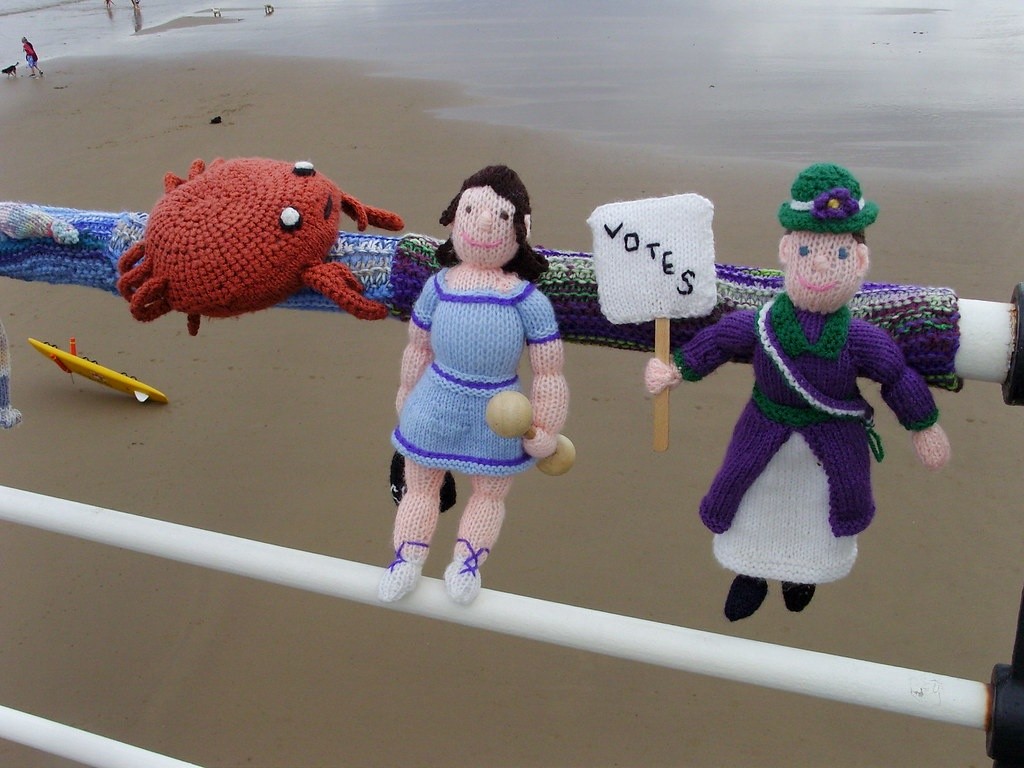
[33,52,38,61]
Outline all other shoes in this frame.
[40,71,43,77]
[29,74,36,77]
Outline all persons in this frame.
[642,163,953,623]
[131,0,139,8]
[104,0,111,8]
[133,9,142,32]
[376,166,570,605]
[22,37,43,77]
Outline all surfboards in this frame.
[29,336,170,405]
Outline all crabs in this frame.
[114,154,414,326]
